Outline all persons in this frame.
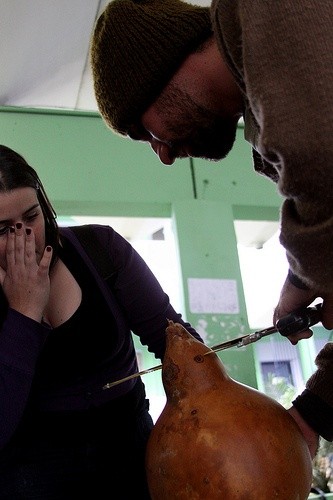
[89,0,333,467]
[1,143,206,498]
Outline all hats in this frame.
[91,1,214,136]
